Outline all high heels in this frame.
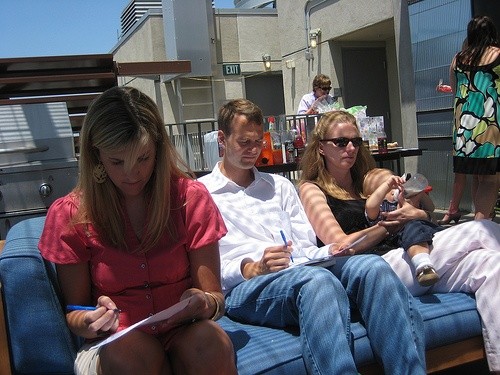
[437,210,461,225]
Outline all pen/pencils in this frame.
[280,230,293,262]
[67,305,121,312]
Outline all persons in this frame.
[363,168,443,287]
[297,74,332,115]
[38,86,237,375]
[296,110,500,375]
[438,16,500,224]
[195,99,426,375]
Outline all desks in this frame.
[374,147,427,175]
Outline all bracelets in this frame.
[205,292,221,320]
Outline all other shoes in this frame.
[415,265,439,287]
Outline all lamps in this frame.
[309,28,321,49]
[262,53,271,69]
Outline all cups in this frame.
[378,137,387,153]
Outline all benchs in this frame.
[0,215,485,375]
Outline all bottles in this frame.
[285,131,304,162]
[268,117,280,149]
[390,173,428,202]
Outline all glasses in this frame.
[320,137,362,148]
[317,87,332,91]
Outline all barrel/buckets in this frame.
[203,131,223,169]
